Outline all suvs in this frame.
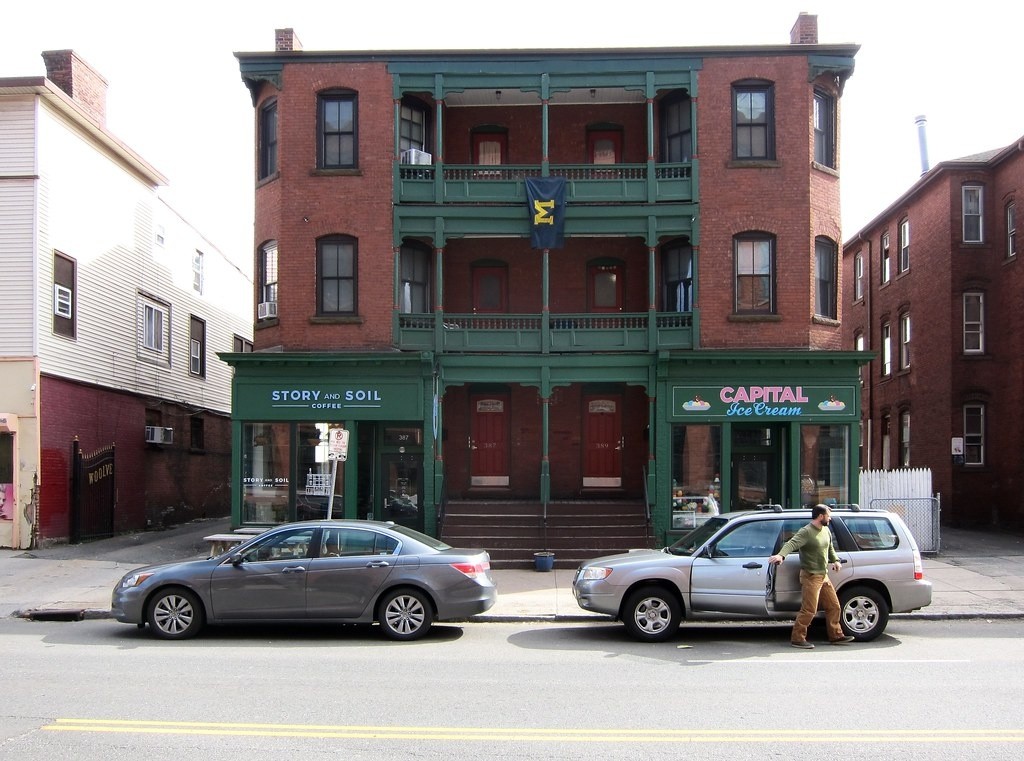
[570,503,932,641]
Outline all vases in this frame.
[534,552,555,572]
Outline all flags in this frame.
[526,176,568,250]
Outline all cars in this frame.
[111,518,498,640]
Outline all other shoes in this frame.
[832,636,855,644]
[792,642,815,649]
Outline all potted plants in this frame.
[306,426,321,445]
[254,432,268,445]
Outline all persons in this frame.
[768,504,855,648]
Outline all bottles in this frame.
[673,477,720,513]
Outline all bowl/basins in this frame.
[534,552,555,571]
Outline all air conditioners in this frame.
[258,303,277,319]
[401,149,431,173]
[145,426,173,444]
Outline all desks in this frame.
[204,528,270,559]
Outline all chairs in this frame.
[326,537,341,556]
